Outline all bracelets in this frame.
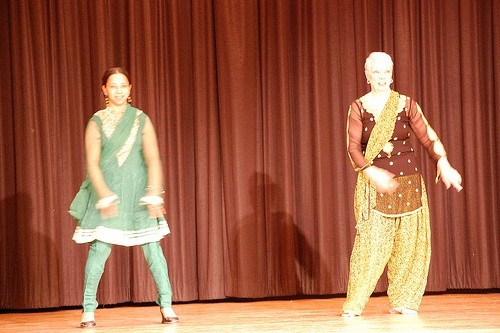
[95,194,121,209]
[139,185,165,206]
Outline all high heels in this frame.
[159,307,179,323]
[80,310,96,327]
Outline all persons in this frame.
[340,52,465,319]
[67,66,180,328]
